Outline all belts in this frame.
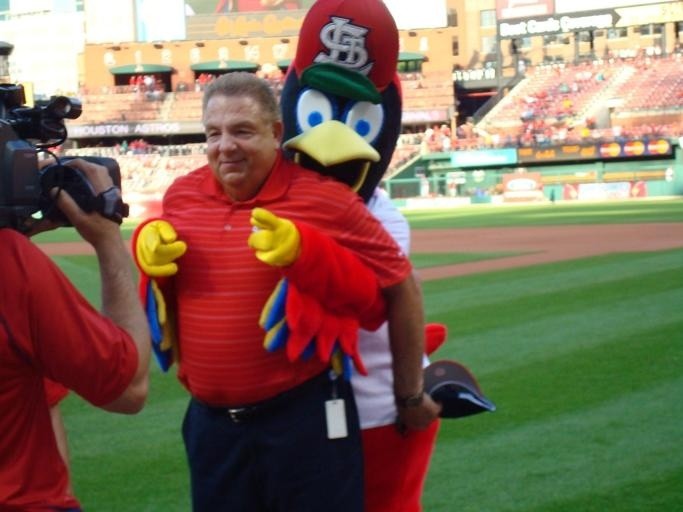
[194,369,330,423]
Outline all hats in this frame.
[423,359,496,417]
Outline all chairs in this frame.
[468,57,683,137]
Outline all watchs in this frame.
[395,393,426,409]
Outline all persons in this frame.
[0,156,148,511]
[46,379,69,464]
[519,58,612,120]
[38,70,288,161]
[158,67,441,508]
[425,117,683,153]
